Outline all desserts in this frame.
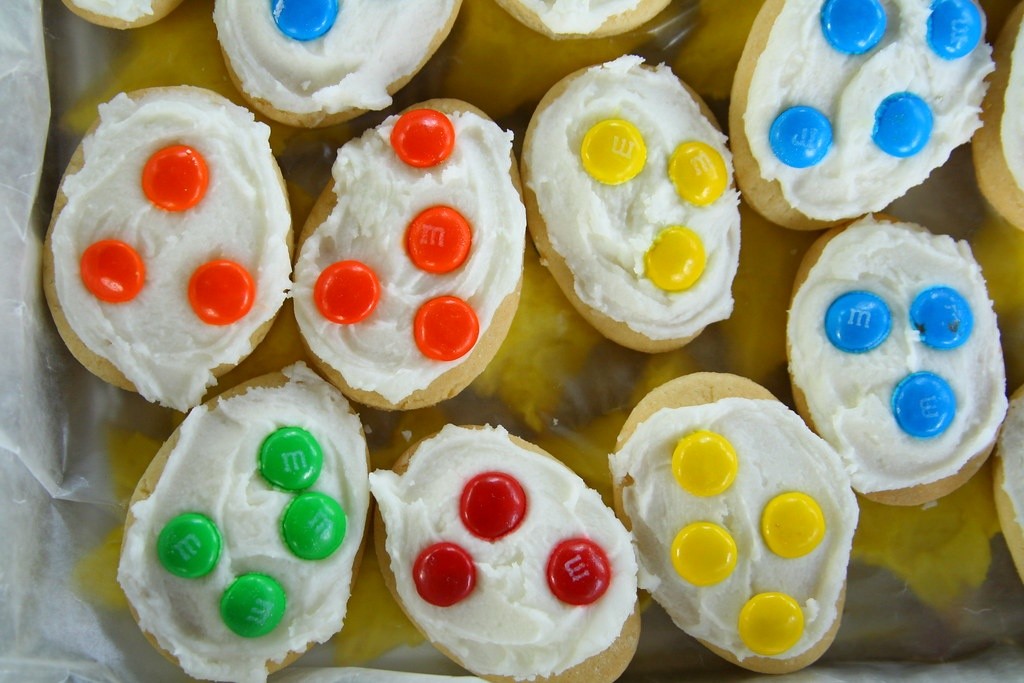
[40,0,1024,683]
